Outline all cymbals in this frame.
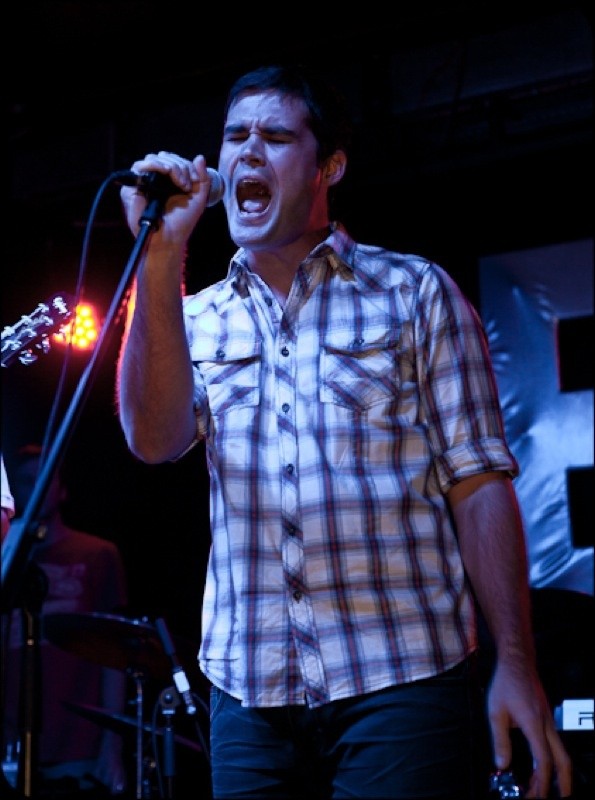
[66,697,204,762]
[44,607,186,678]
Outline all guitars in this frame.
[0,287,75,371]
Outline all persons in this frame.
[114,66,579,800]
[1,444,129,800]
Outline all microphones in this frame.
[113,166,225,208]
